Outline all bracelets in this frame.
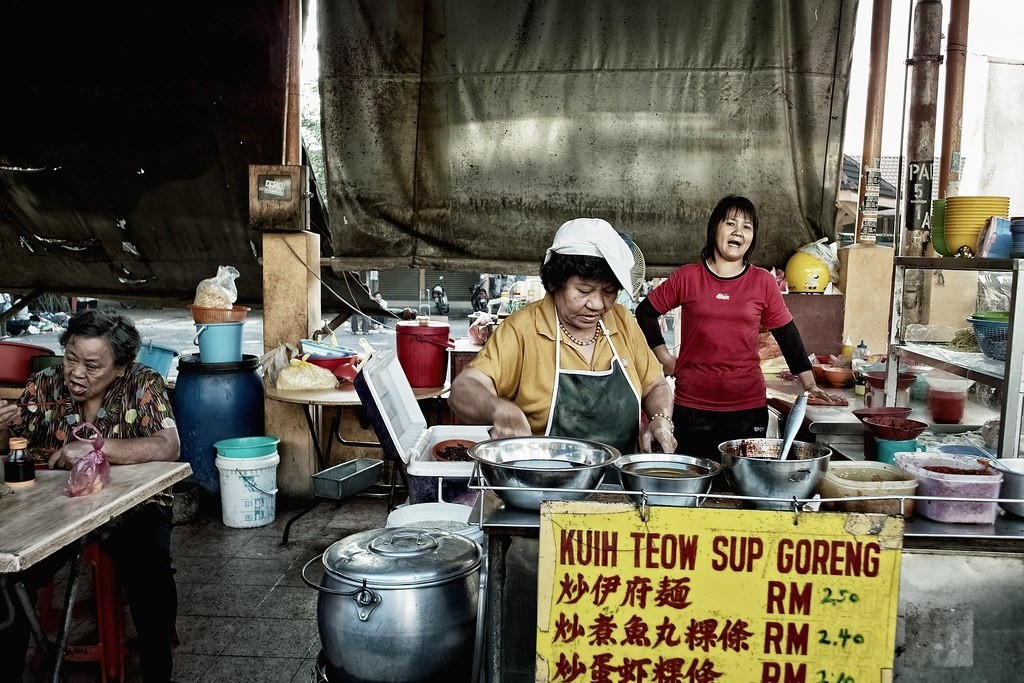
[649,412,674,434]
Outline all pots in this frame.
[300,526,482,683]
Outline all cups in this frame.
[864,429,926,465]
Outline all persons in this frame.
[446,218,677,485]
[634,193,846,491]
[0,308,180,683]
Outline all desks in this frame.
[266,373,443,544]
[0,455,194,683]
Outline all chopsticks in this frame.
[11,402,66,405]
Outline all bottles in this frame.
[497,290,535,325]
[841,336,868,396]
[4,437,35,490]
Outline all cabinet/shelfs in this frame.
[883,256,1024,457]
[450,338,484,388]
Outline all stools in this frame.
[30,542,179,683]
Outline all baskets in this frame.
[967,317,1010,361]
[187,304,251,324]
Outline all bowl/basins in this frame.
[296,339,358,379]
[610,453,722,507]
[467,437,620,511]
[432,440,477,462]
[989,458,1024,517]
[931,196,1011,258]
[187,304,251,324]
[811,356,976,440]
[718,438,832,509]
[0,341,55,383]
[213,436,280,458]
[971,311,1010,334]
[30,355,63,373]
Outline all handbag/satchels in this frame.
[193,266,240,310]
[62,423,111,496]
[276,354,339,391]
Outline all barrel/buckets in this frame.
[194,320,245,363]
[384,502,484,548]
[396,321,457,388]
[215,449,280,528]
[173,354,265,496]
[135,339,179,379]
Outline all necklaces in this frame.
[559,322,600,346]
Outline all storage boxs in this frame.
[310,456,384,500]
[352,349,494,505]
[821,461,918,517]
[891,451,1004,524]
